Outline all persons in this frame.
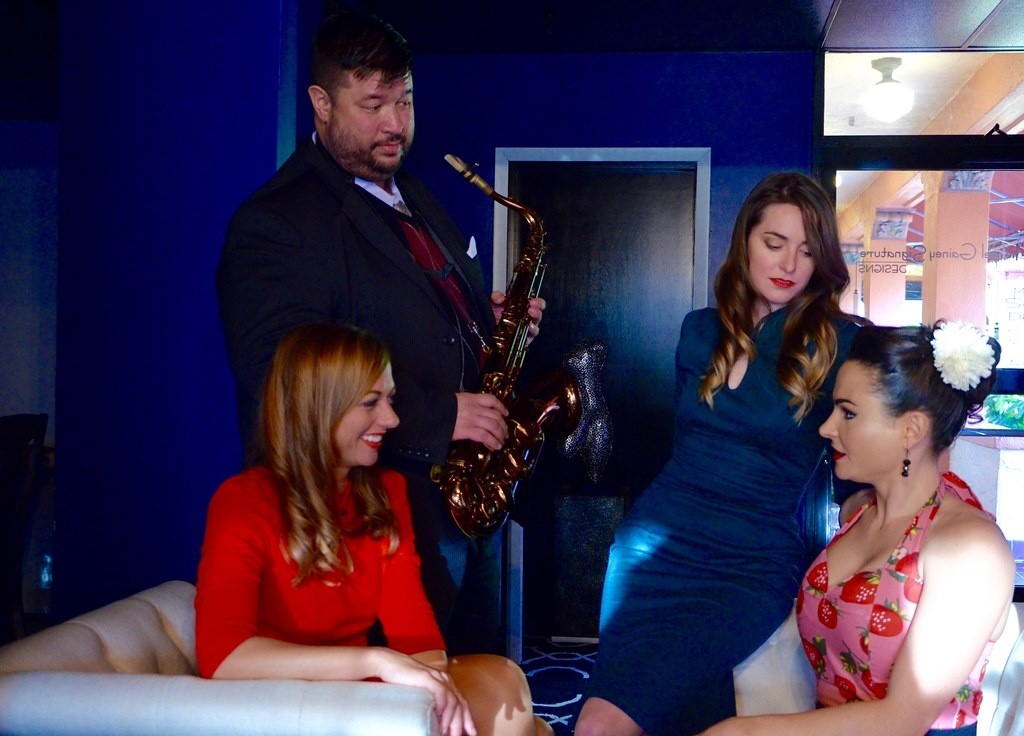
[193,319,533,736]
[217,18,548,656]
[571,171,874,736]
[699,317,1016,736]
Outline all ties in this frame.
[392,199,412,216]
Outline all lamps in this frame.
[861,58,914,122]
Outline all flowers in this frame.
[929,318,996,392]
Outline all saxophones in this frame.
[435,151,590,545]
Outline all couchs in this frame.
[0,581,441,736]
[733,598,1024,736]
